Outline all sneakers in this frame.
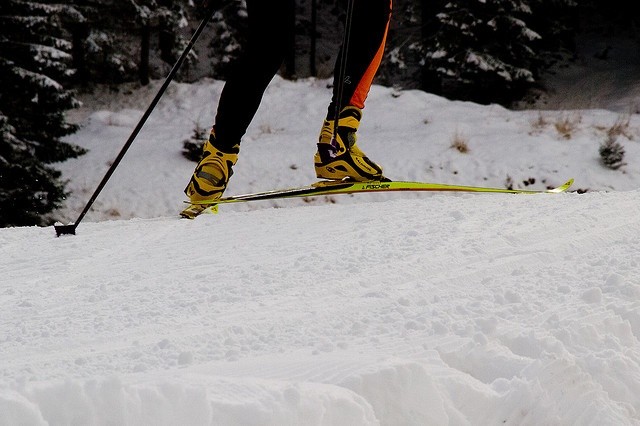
[314,106,382,182]
[184,128,240,203]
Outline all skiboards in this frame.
[180,177,576,218]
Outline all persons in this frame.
[185,1,393,200]
